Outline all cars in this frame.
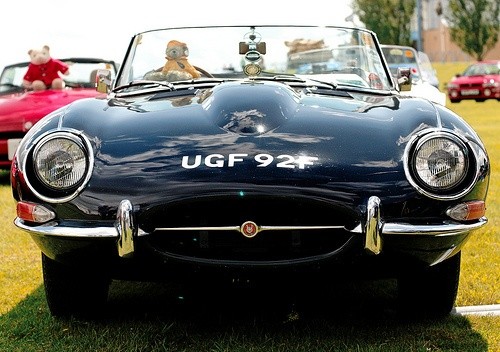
[446,61,500,103]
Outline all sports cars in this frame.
[379,43,447,107]
[10,22,491,320]
[0,57,119,184]
